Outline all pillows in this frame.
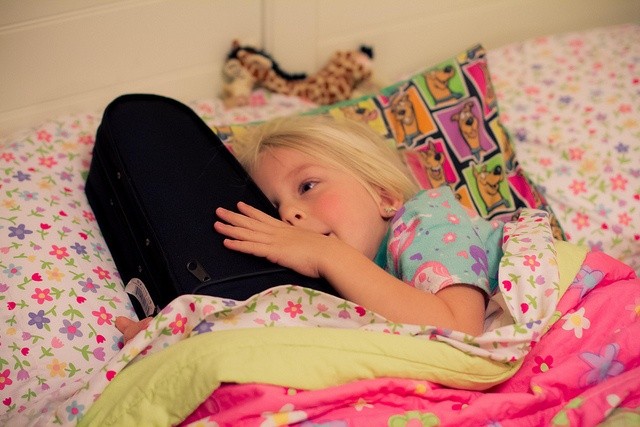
[1,87,330,423]
[477,24,639,261]
[208,41,569,242]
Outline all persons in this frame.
[114,113,507,348]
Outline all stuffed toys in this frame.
[221,39,374,107]
